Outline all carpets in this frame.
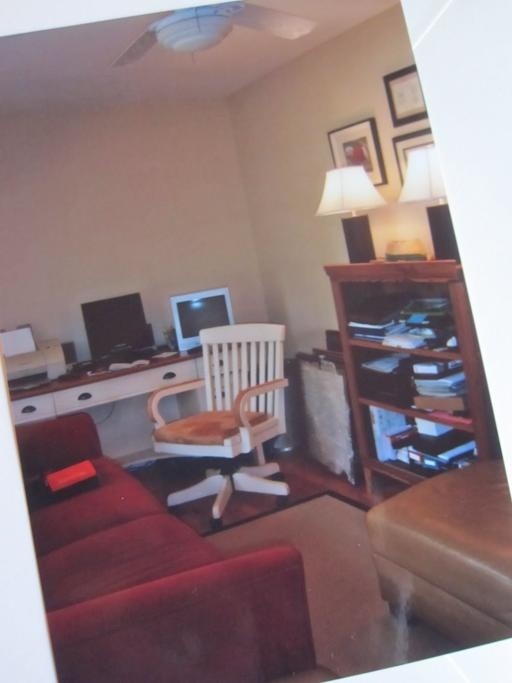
[200,491,471,679]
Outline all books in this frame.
[348,314,409,340]
[409,356,465,398]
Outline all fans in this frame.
[113,2,316,67]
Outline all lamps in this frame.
[315,164,386,263]
[397,144,461,260]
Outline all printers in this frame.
[0,323,78,391]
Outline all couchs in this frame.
[14,412,316,683]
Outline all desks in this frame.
[9,348,250,426]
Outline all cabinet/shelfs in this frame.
[323,258,503,495]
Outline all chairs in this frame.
[147,323,290,530]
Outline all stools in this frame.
[366,459,512,648]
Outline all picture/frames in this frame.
[391,126,436,188]
[326,116,387,187]
[382,63,429,128]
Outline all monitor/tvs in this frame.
[169,287,235,352]
[81,292,151,360]
